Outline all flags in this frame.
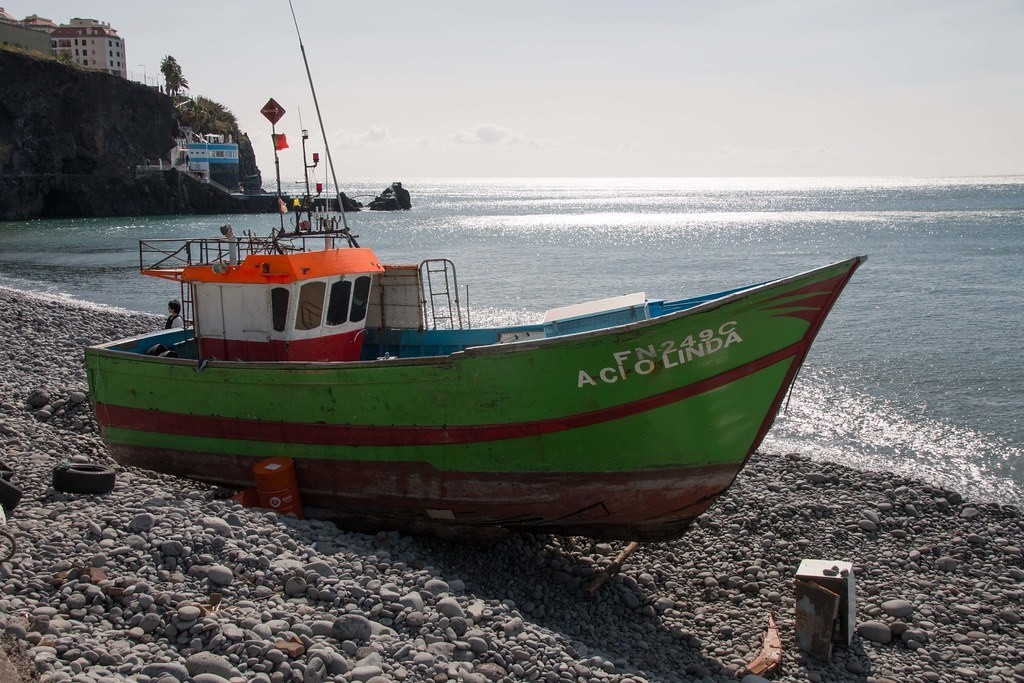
[272,134,289,150]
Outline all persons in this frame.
[165,301,183,328]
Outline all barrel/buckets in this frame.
[252,457,304,520]
[228,488,261,509]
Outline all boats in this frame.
[82,98,869,541]
